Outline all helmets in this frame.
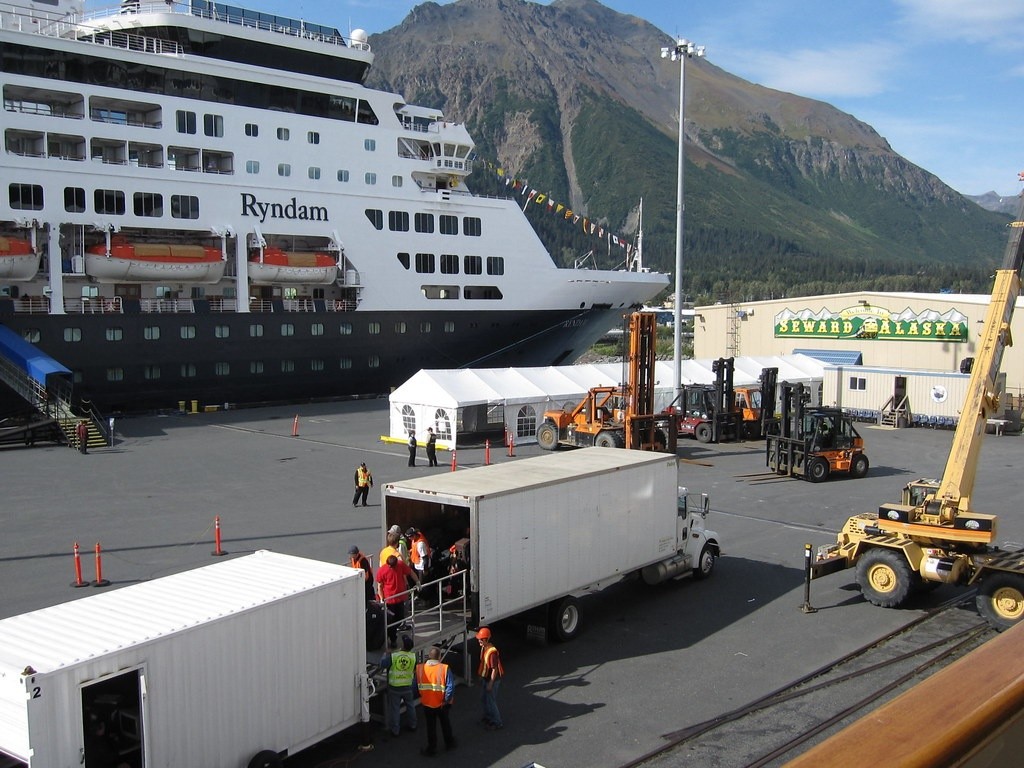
[476,628,491,638]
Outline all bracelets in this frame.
[415,579,422,585]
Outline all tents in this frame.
[388,353,837,450]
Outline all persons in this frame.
[352,463,373,507]
[381,634,416,737]
[347,545,375,609]
[416,648,455,759]
[84,719,130,768]
[408,430,417,467]
[916,488,929,505]
[76,420,89,454]
[426,428,437,467]
[476,628,504,729]
[373,555,422,648]
[379,524,435,606]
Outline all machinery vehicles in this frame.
[799,171,1023,633]
[660,358,743,443]
[733,367,782,441]
[733,381,869,483]
[536,312,678,454]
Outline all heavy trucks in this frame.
[1,446,722,767]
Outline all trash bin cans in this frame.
[179,401,185,412]
[191,400,198,412]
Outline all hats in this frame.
[361,462,365,466]
[388,525,401,533]
[347,545,358,554]
[405,527,420,537]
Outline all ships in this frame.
[0,0,670,418]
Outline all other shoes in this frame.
[397,625,411,631]
[391,641,397,648]
[486,721,504,729]
[478,715,490,725]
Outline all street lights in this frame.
[660,38,706,408]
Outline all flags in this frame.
[470,154,639,260]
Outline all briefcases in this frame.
[455,538,471,572]
